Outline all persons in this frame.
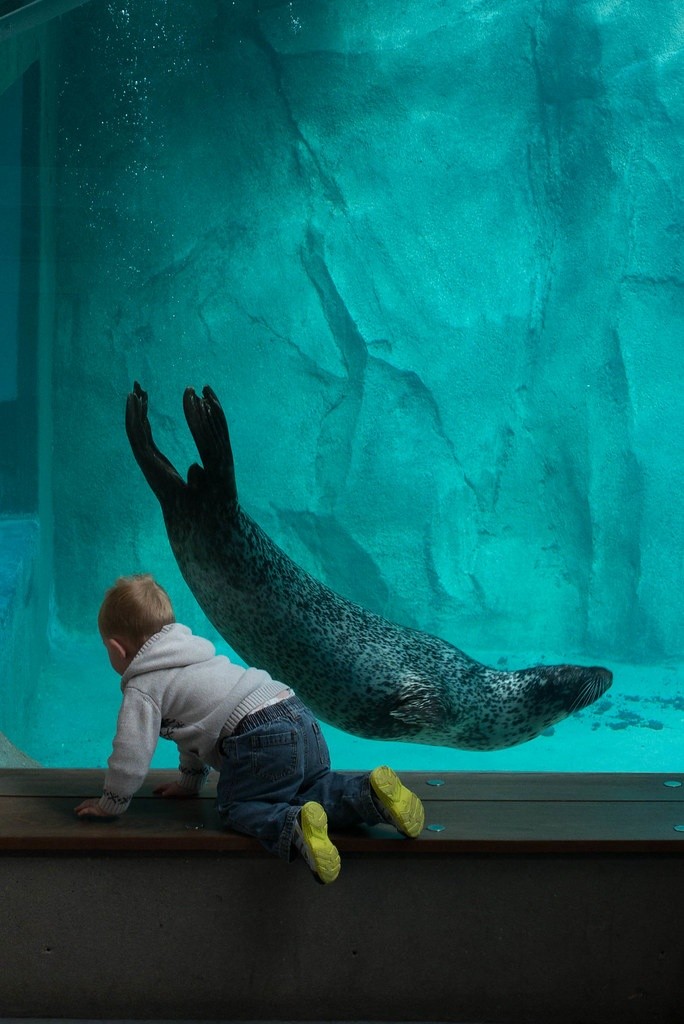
[75,575,424,885]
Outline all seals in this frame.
[124,380,613,751]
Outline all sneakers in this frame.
[291,801,341,886]
[369,765,425,839]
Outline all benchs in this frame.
[0,767,684,1023]
